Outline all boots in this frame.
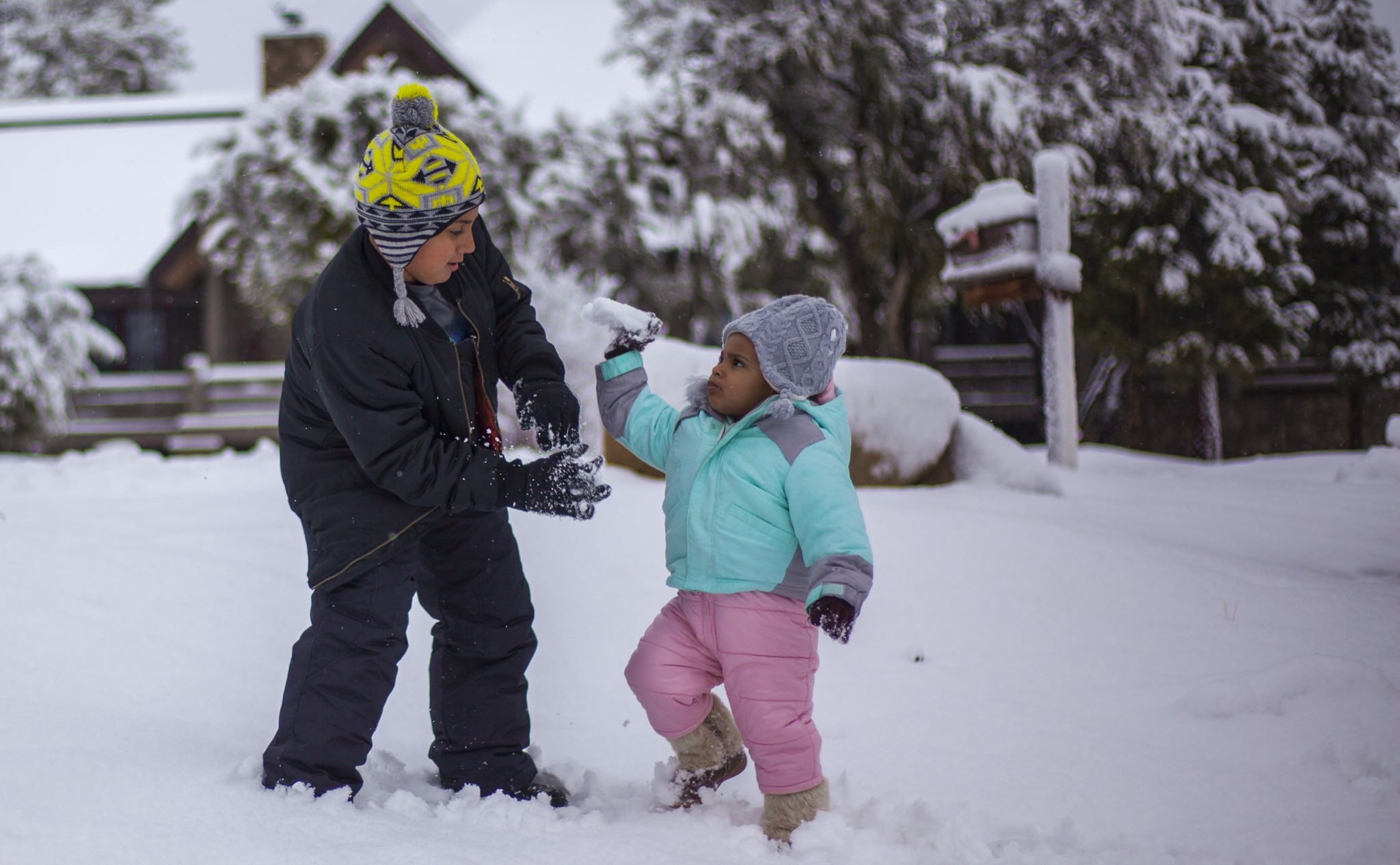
[759,781,829,845]
[661,696,747,810]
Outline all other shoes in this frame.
[524,771,573,808]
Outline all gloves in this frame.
[507,445,611,519]
[514,383,579,451]
[603,313,663,359]
[808,596,856,643]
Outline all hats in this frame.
[352,86,486,329]
[688,294,848,420]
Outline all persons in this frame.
[595,292,876,842]
[264,81,611,807]
